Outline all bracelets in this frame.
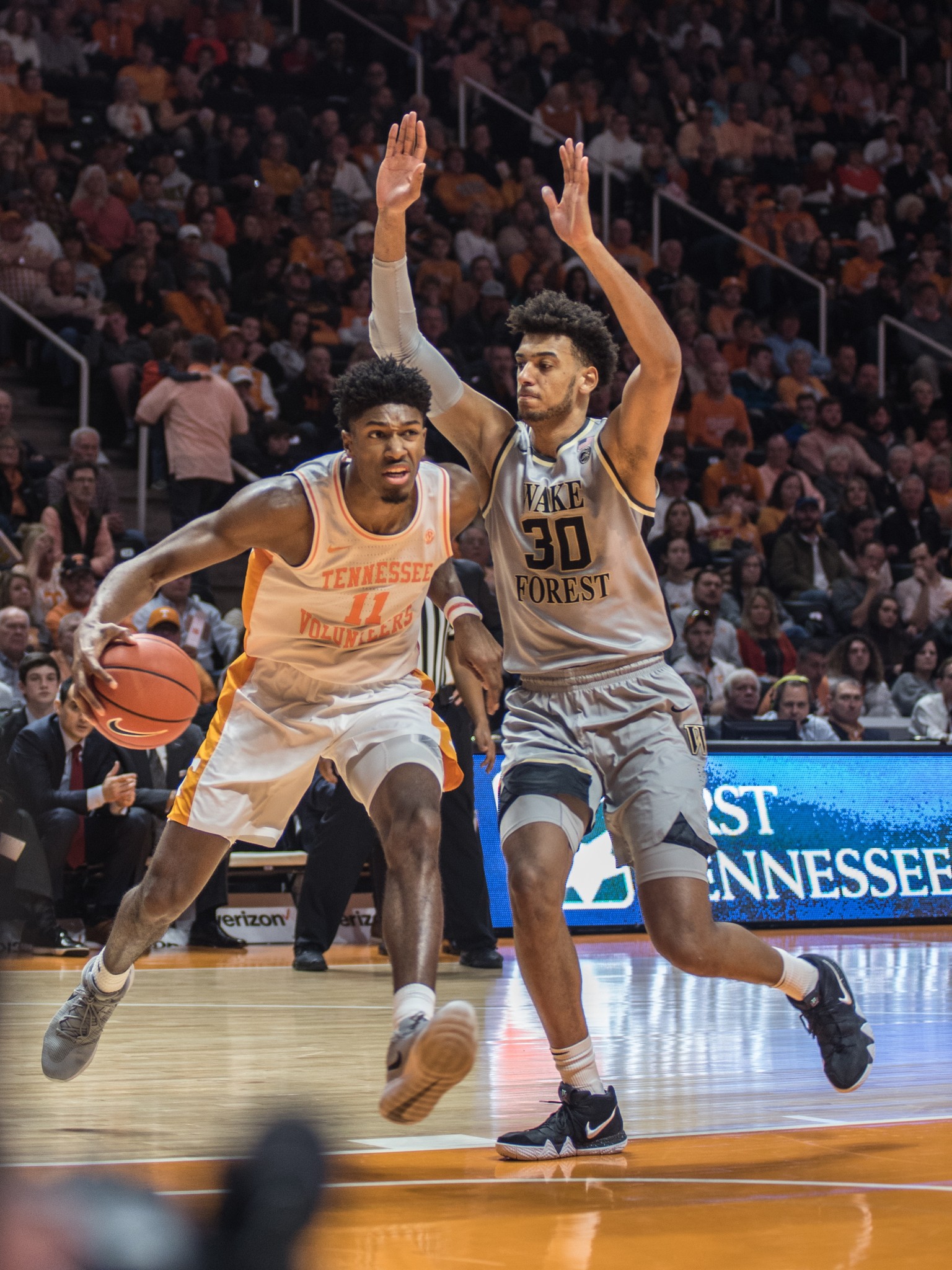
[444,597,483,628]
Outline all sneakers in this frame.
[41,954,135,1083]
[495,1082,628,1161]
[785,953,875,1093]
[31,926,90,957]
[379,1000,479,1126]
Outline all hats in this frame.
[58,552,94,577]
[149,607,180,628]
[794,495,818,508]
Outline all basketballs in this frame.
[90,632,202,748]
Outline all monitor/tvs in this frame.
[718,719,801,741]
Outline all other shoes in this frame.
[188,916,246,949]
[460,948,503,969]
[292,945,328,971]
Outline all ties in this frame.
[67,744,85,874]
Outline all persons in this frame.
[0,0,952,1270]
[368,110,878,1160]
